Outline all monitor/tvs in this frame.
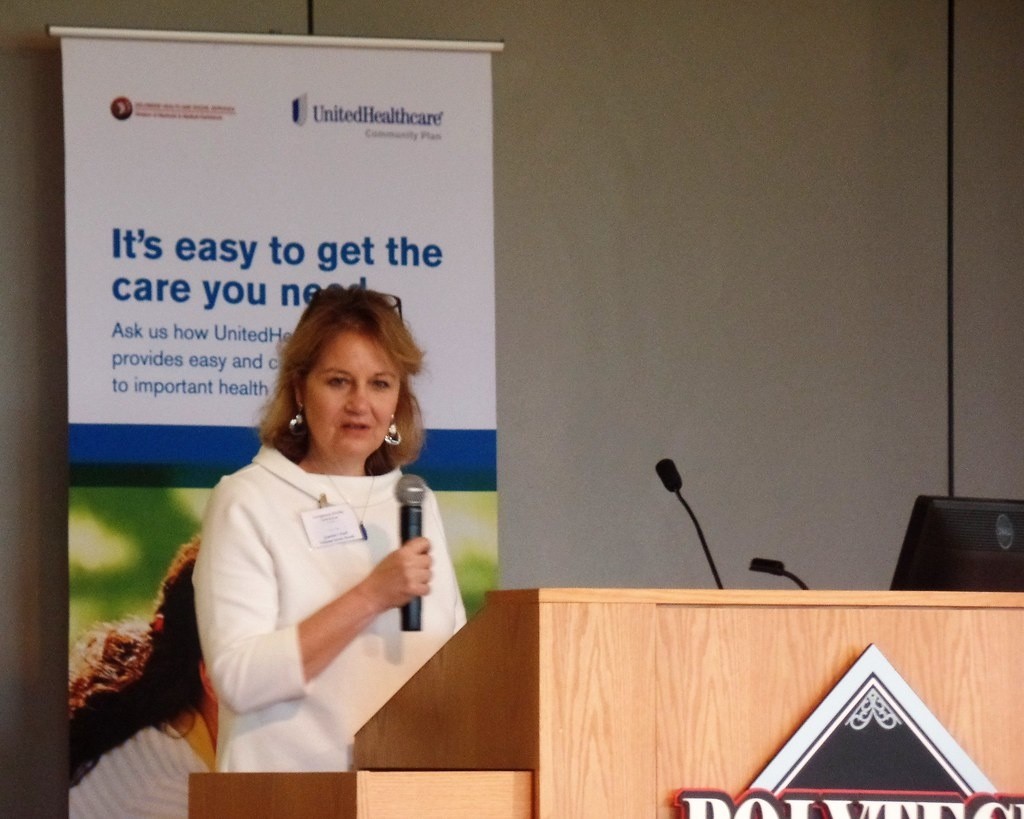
[889,494,1024,593]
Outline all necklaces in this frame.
[303,451,376,540]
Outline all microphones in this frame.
[655,459,725,591]
[395,474,425,631]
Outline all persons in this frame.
[191,286,467,773]
[68,531,219,819]
